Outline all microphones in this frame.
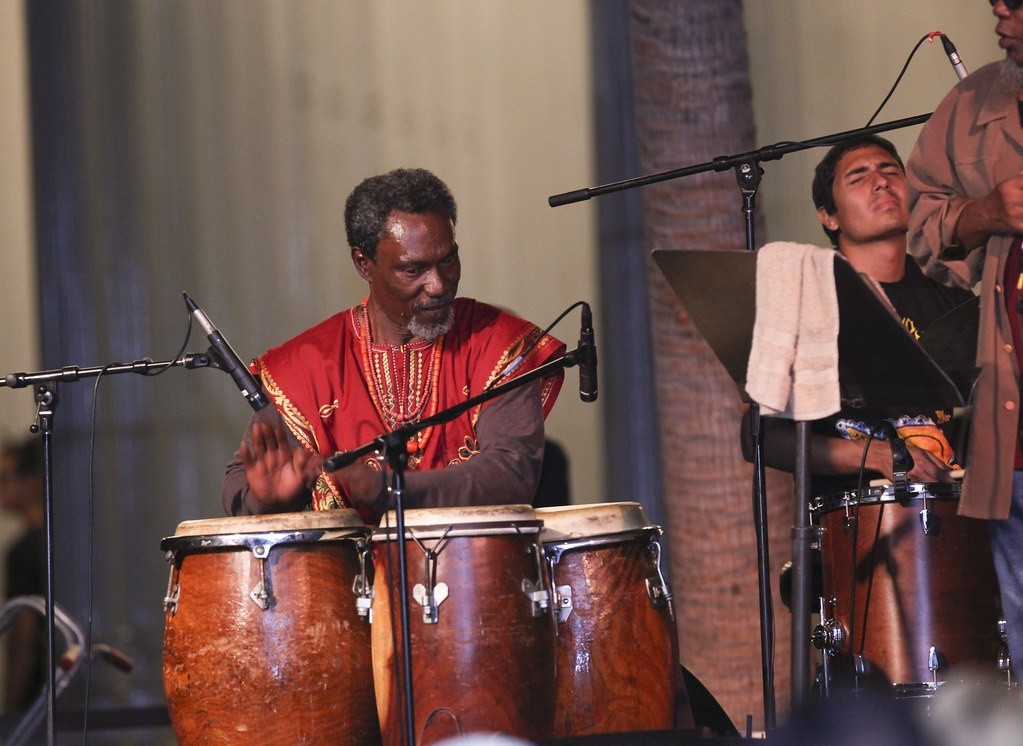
[186,296,270,413]
[885,422,916,474]
[581,303,599,402]
[942,34,970,81]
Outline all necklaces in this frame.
[360,292,455,453]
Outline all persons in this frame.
[740,135,979,526]
[901,0,1023,677]
[0,440,47,725]
[219,169,568,513]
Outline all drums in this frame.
[370,505,557,746]
[162,510,379,745]
[534,501,679,736]
[810,483,1007,686]
